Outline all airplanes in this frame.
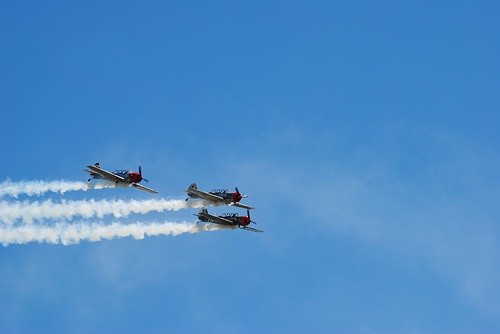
[185,182,254,211]
[191,207,264,233]
[82,162,159,194]
